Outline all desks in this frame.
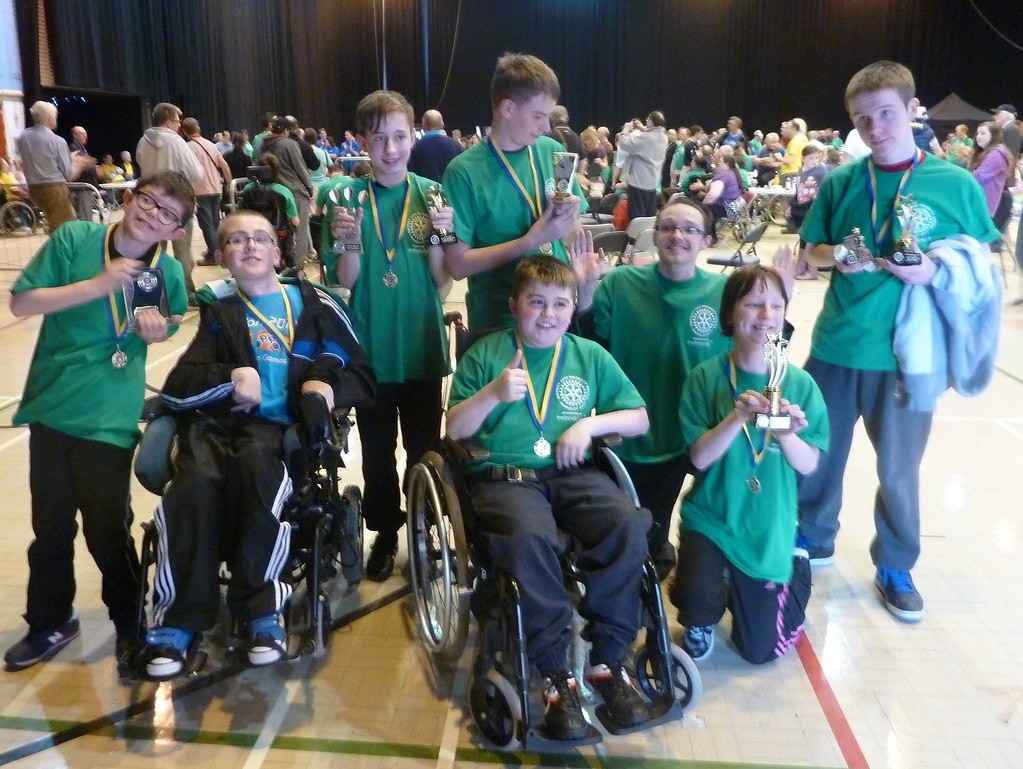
[99,179,136,223]
[748,187,796,226]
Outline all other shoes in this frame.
[781,228,797,235]
[197,259,218,266]
[201,251,210,258]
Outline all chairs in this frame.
[989,189,1017,288]
[689,173,769,274]
[781,172,798,188]
[574,194,657,279]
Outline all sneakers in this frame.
[425,535,436,577]
[682,622,717,662]
[540,671,588,738]
[144,623,195,677]
[4,605,80,667]
[246,610,285,667]
[111,609,148,666]
[794,534,834,567]
[872,566,924,620]
[365,533,399,582]
[580,650,649,726]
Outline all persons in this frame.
[5,171,196,667]
[569,196,795,583]
[446,255,652,740]
[147,213,377,676]
[797,61,1001,619]
[668,263,830,665]
[442,52,589,331]
[319,90,453,581]
[911,105,1023,270]
[407,105,871,279]
[0,101,373,275]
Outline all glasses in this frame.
[167,117,183,125]
[653,224,706,239]
[221,234,276,252]
[131,189,182,228]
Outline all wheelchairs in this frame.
[125,283,365,675]
[404,311,702,754]
[0,174,36,236]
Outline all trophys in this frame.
[329,187,369,254]
[552,152,578,202]
[424,186,458,249]
[890,194,922,266]
[842,227,873,263]
[755,332,790,430]
[121,267,172,330]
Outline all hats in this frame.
[271,116,291,130]
[989,104,1017,115]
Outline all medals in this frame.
[112,352,127,368]
[539,242,552,254]
[873,259,882,271]
[749,478,760,493]
[383,272,397,287]
[534,439,551,458]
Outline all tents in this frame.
[926,93,995,144]
